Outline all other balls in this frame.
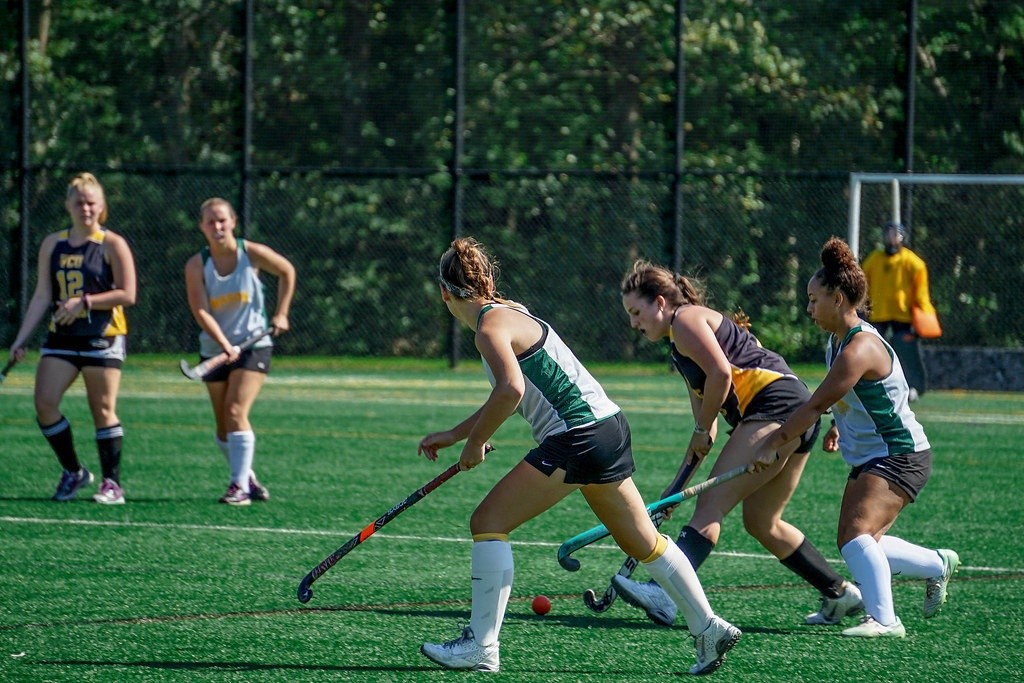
[530,594,552,615]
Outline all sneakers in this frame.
[219,483,252,506]
[611,574,679,626]
[690,614,742,675]
[92,478,125,505]
[841,614,906,638]
[420,622,499,672]
[805,581,864,625]
[248,478,267,499]
[924,549,961,617]
[51,465,93,501]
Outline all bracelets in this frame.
[80,292,92,324]
[830,419,836,426]
[693,424,709,435]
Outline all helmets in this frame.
[883,223,906,255]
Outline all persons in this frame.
[610,258,864,629]
[858,220,933,402]
[746,235,960,639]
[415,233,743,676]
[9,173,138,506]
[185,197,296,506]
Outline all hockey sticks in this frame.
[179,328,274,380]
[0,354,23,386]
[584,457,705,613]
[556,467,745,572]
[298,441,496,604]
